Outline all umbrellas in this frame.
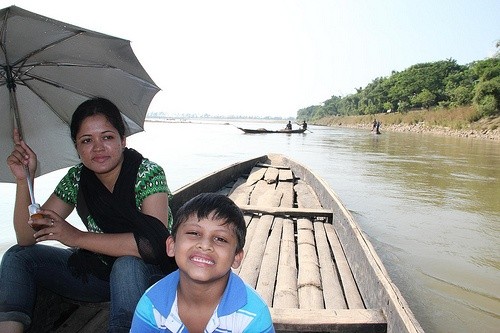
[0,3,163,228]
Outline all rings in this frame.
[50,218,55,226]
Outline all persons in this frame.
[372,119,377,131]
[285,121,292,130]
[129,193,276,333]
[300,121,307,130]
[0,97,173,333]
[375,123,381,134]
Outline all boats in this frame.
[236,124,306,133]
